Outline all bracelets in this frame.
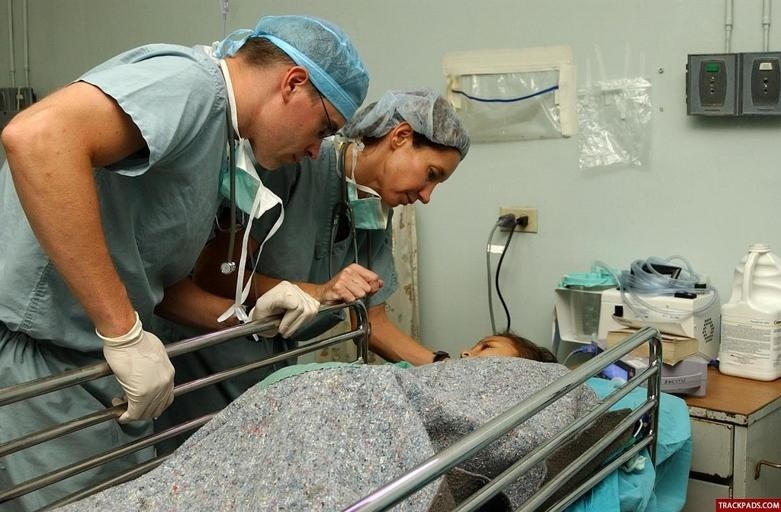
[432,348,451,364]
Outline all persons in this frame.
[459,331,561,367]
[1,13,370,510]
[149,88,473,452]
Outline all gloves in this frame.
[251,279,322,340]
[94,310,178,425]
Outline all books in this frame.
[606,315,701,365]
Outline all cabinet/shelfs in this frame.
[566,362,781,512]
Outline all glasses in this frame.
[318,89,336,140]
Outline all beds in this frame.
[2,299,690,512]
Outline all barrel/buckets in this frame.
[719,244,781,382]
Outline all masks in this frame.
[334,134,391,232]
[217,59,286,328]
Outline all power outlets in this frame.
[498,206,538,234]
